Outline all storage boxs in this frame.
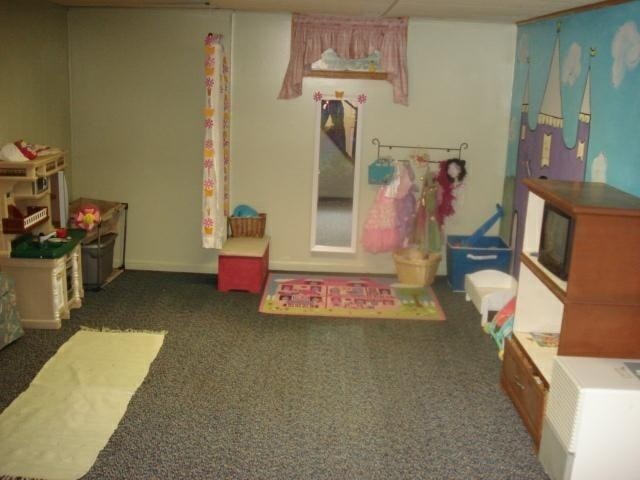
[80,233,117,289]
[443,232,511,295]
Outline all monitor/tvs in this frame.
[538,201,571,281]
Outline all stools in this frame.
[216,235,273,294]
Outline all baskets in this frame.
[68,198,123,244]
[393,246,441,286]
[228,212,266,238]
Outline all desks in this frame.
[0,145,86,330]
[65,198,130,295]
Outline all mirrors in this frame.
[308,93,360,254]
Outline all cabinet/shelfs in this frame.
[496,182,640,446]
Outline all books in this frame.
[529,331,561,348]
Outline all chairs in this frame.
[536,354,640,480]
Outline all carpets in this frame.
[254,270,448,323]
[2,323,171,479]
